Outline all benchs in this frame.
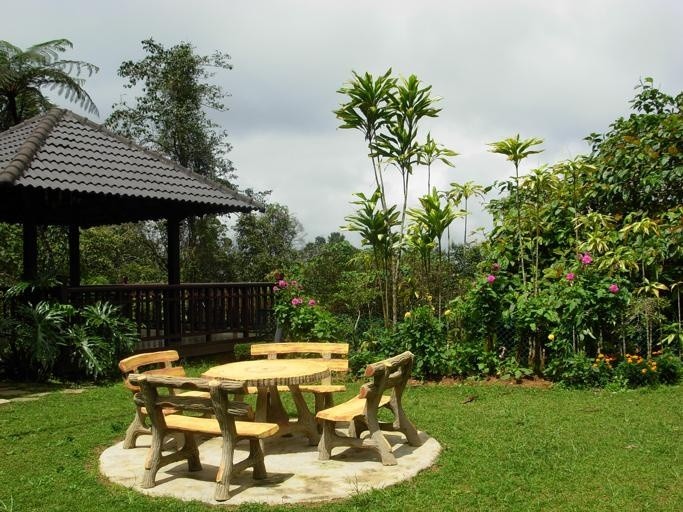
[128,373,279,501]
[118,350,211,451]
[251,342,349,433]
[315,351,421,465]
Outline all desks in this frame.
[202,360,330,446]
